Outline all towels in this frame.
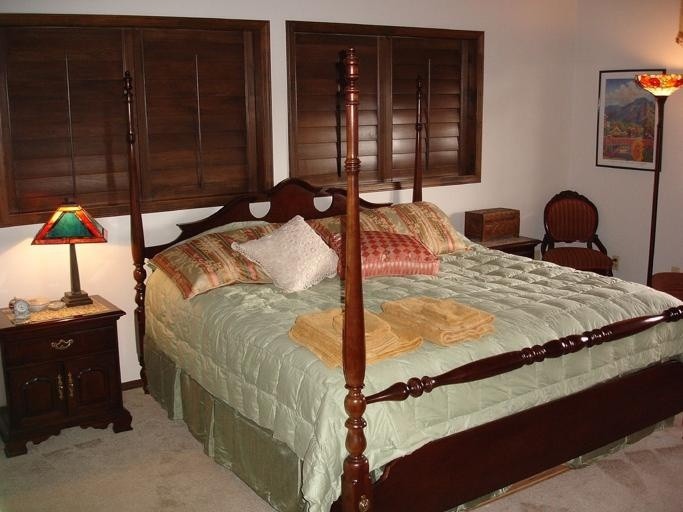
[288,294,498,372]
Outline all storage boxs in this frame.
[464,208,520,242]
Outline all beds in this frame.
[140,178,683,512]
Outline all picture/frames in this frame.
[595,68,666,172]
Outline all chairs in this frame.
[542,191,613,276]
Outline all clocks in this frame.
[13,300,30,319]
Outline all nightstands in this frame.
[0,295,134,458]
[465,235,541,259]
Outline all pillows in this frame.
[304,201,465,256]
[231,214,339,293]
[152,223,287,299]
[329,231,440,280]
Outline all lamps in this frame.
[30,203,109,308]
[635,73,683,288]
